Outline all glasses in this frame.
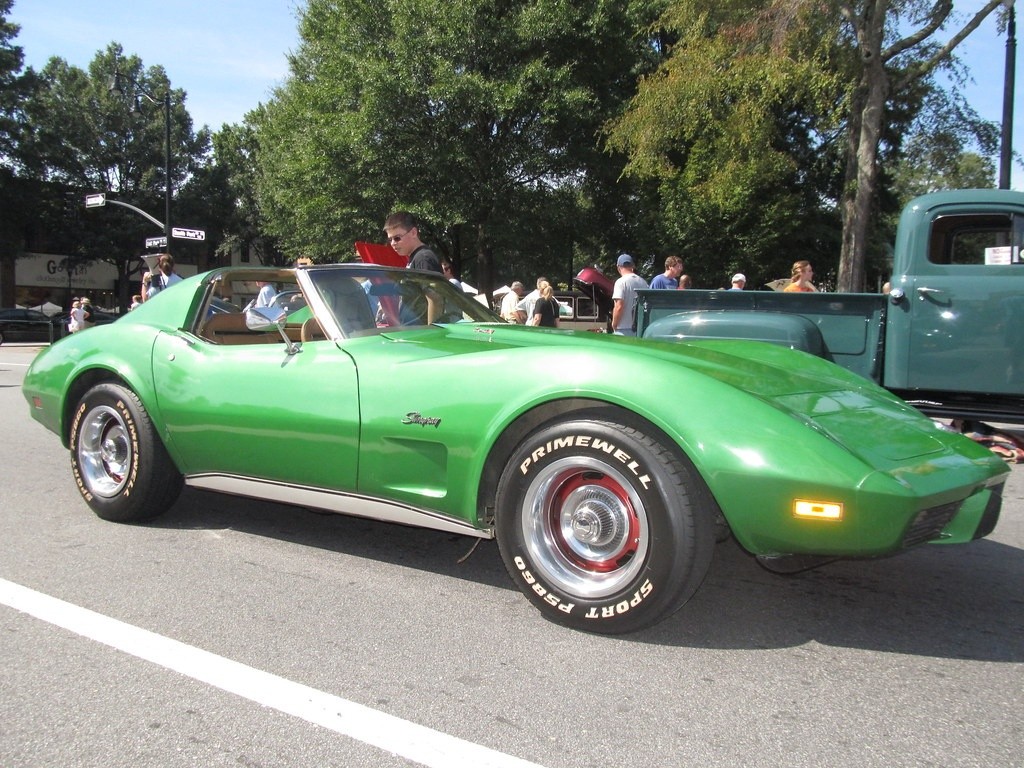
[443,265,452,273]
[387,225,416,244]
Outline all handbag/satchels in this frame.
[68,318,79,332]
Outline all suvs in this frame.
[243,290,308,315]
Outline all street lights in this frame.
[111,70,176,254]
[998,5,1017,190]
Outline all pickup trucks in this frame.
[632,186,1024,430]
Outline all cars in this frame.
[21,261,1011,636]
[51,311,119,341]
[0,308,62,345]
[494,290,612,332]
[207,296,242,319]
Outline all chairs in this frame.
[203,315,328,344]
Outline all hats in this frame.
[81,298,90,304]
[616,253,632,266]
[73,297,79,300]
[732,273,747,284]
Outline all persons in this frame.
[783,260,815,292]
[141,254,184,302]
[131,295,141,310]
[255,281,276,307]
[440,261,463,321]
[677,274,693,289]
[70,297,96,333]
[501,277,561,327]
[361,279,379,319]
[649,256,684,290]
[728,273,746,290]
[382,211,444,326]
[611,254,649,336]
